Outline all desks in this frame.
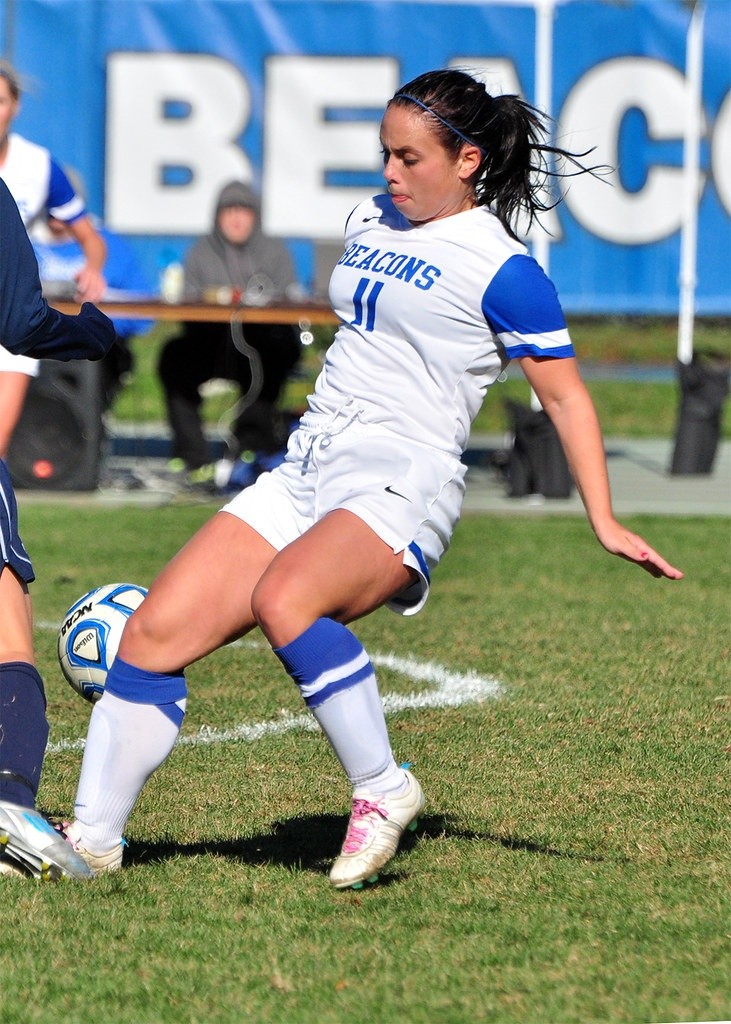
[45,300,361,460]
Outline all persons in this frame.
[60,71,689,882]
[0,66,138,474]
[153,181,313,497]
[0,146,134,891]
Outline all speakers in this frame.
[3,355,107,492]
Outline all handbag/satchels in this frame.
[507,410,573,498]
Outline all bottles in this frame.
[158,260,185,306]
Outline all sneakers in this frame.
[329,762,425,889]
[0,822,129,880]
[0,799,94,883]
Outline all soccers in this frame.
[56,582,151,705]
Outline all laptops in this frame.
[305,240,348,306]
